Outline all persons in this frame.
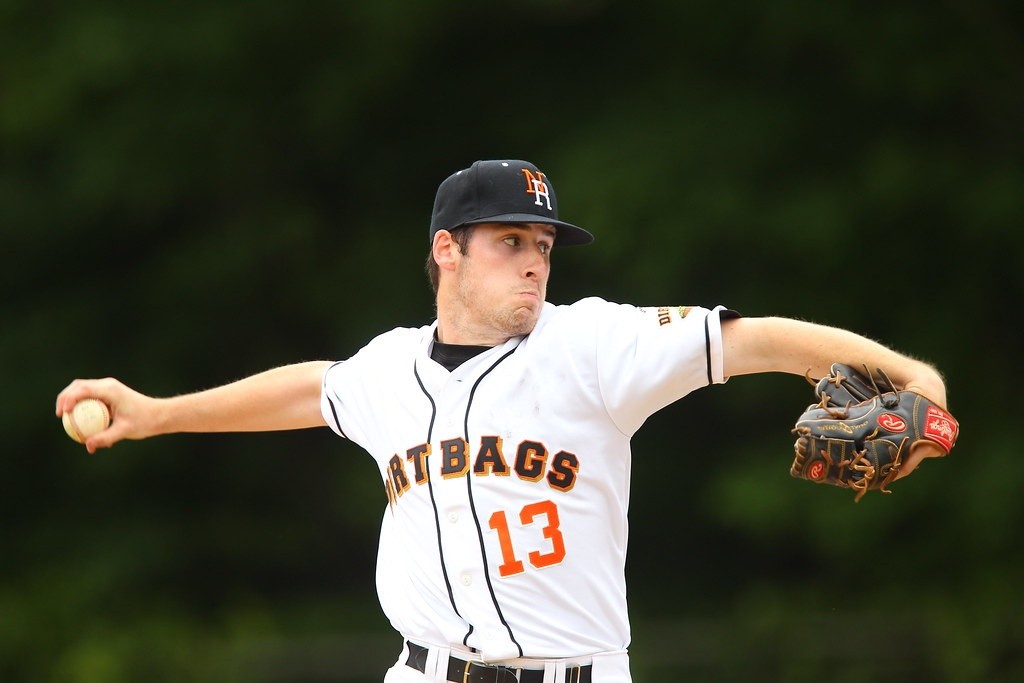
[57,160,960,683]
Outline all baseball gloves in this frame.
[788,361,957,496]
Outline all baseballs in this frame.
[63,397,109,444]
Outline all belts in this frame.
[405,641,593,683]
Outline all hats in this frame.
[429,159,594,245]
